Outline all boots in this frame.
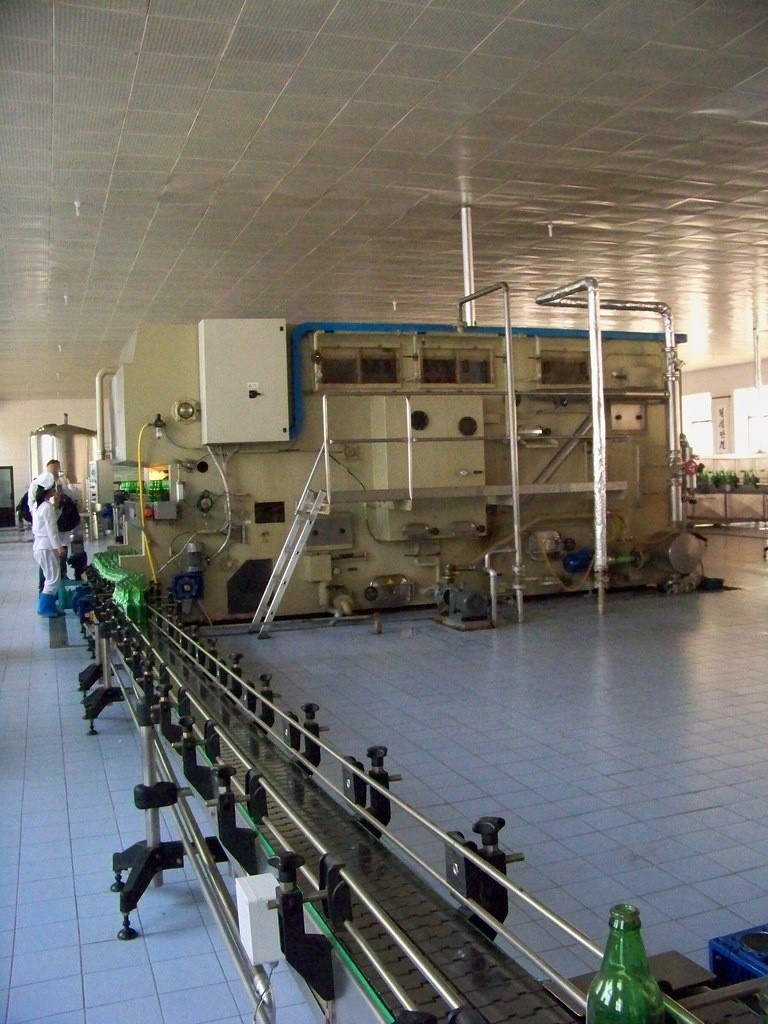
[36,592,67,619]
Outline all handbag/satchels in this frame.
[15,492,31,522]
[57,496,80,532]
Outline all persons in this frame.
[27,460,75,598]
[32,473,67,618]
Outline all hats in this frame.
[35,472,55,490]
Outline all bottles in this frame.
[586,904,664,1024]
[750,473,756,487]
[113,477,170,502]
[91,545,146,625]
[743,472,750,485]
[696,470,738,491]
